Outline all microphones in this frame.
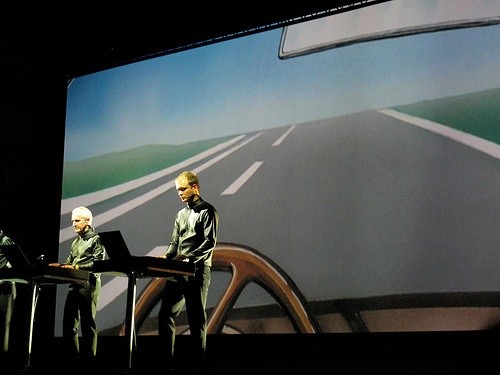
[33,255,44,263]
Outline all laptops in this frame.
[97,230,137,259]
[0,244,33,267]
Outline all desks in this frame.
[0,271,90,372]
[91,265,196,369]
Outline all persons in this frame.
[158,171,219,375]
[0,230,30,375]
[48,207,105,375]
